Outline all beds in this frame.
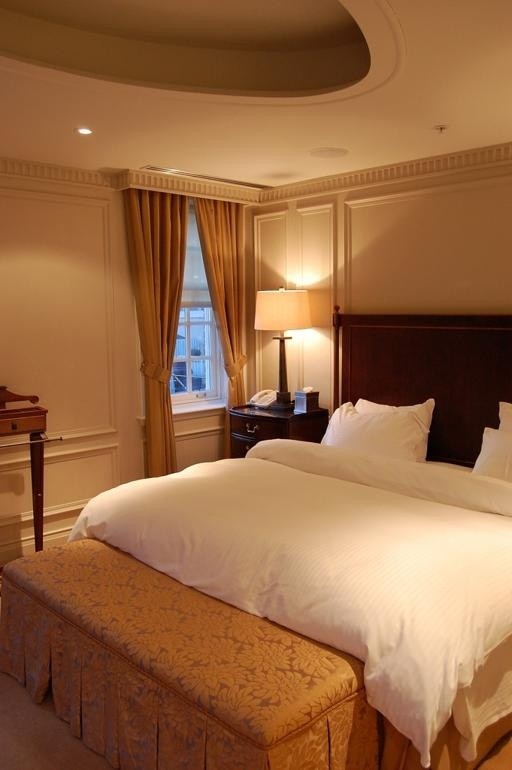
[76,302,511,770]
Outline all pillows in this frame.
[357,392,436,417]
[472,426,512,489]
[319,400,432,461]
[495,400,511,429]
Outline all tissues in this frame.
[294,386,320,414]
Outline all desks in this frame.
[2,433,64,554]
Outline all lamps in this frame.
[248,289,317,409]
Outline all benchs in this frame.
[3,538,384,770]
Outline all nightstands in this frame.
[230,402,323,460]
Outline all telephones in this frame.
[250,390,278,409]
